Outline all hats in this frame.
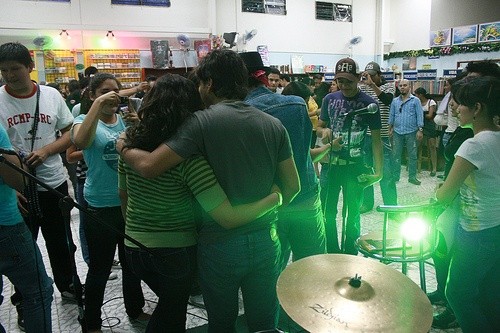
[333,57,361,82]
[364,61,381,75]
[238,52,264,70]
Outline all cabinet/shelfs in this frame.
[29,49,142,98]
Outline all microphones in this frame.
[338,104,378,118]
[0,148,17,155]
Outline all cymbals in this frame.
[276,253,433,333]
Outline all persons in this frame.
[316,56,384,256]
[30,66,152,278]
[433,76,500,333]
[70,71,142,333]
[116,49,301,333]
[388,79,422,186]
[442,76,461,146]
[415,87,437,177]
[0,40,85,331]
[240,52,328,273]
[266,68,341,178]
[118,52,302,333]
[427,59,500,328]
[0,124,55,333]
[358,61,404,223]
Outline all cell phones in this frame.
[119,96,129,104]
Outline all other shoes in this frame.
[430,171,436,176]
[108,271,118,280]
[61,284,85,302]
[189,294,205,308]
[431,306,460,329]
[437,174,445,178]
[112,259,123,269]
[387,211,397,218]
[409,177,421,185]
[360,206,372,214]
[425,289,449,306]
[128,313,152,327]
[16,305,24,330]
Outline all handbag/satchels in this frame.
[16,168,44,220]
[422,111,440,136]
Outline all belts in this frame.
[444,132,453,135]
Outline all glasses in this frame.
[399,104,403,112]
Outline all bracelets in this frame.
[328,141,334,153]
[275,190,282,206]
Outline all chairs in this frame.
[352,198,450,295]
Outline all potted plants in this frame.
[405,49,421,70]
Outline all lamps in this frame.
[223,31,241,49]
[244,28,256,43]
[350,36,363,45]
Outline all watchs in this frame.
[418,128,424,133]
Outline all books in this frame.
[44,52,141,89]
[408,77,449,159]
[269,64,327,74]
